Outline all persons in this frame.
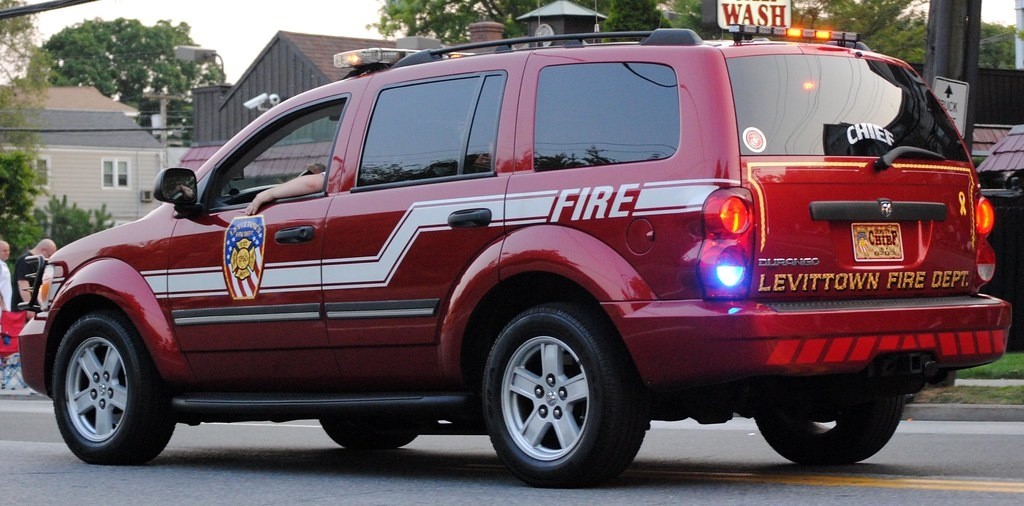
[0,240,13,333]
[11,239,57,323]
[243,162,326,216]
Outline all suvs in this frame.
[15,26,1012,488]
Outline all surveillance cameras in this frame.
[243,94,268,110]
[270,94,280,105]
